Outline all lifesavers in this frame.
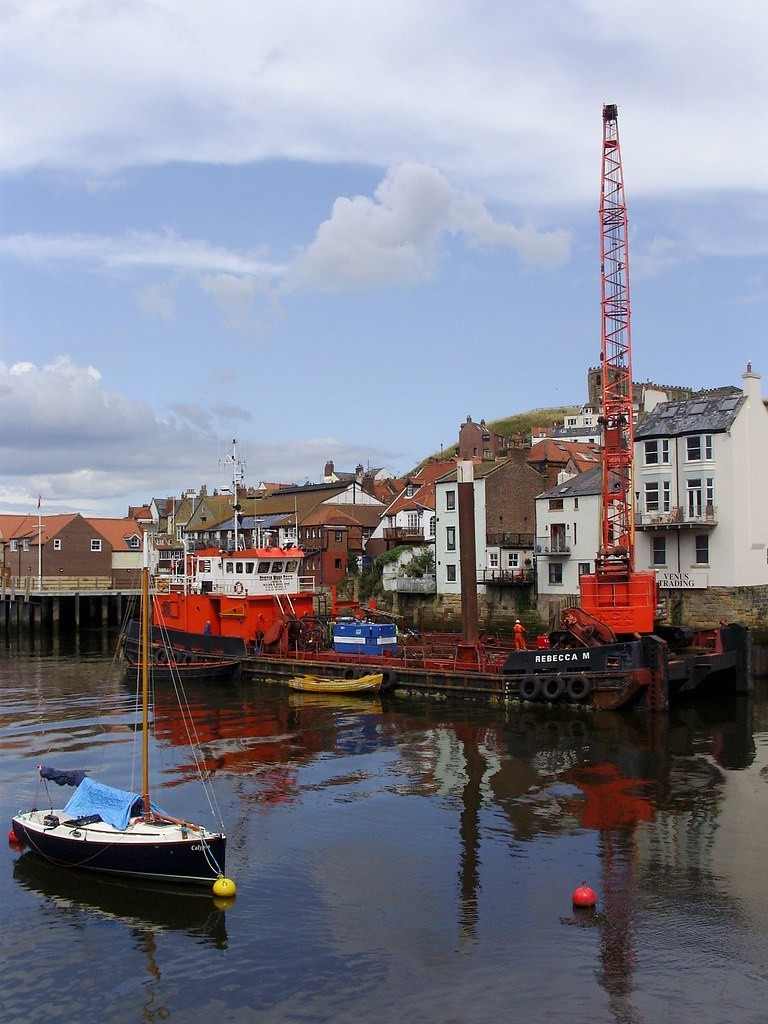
[542,678,565,700]
[182,653,197,663]
[234,582,243,594]
[521,675,540,700]
[358,667,377,679]
[171,651,182,663]
[342,667,359,679]
[379,669,396,689]
[567,675,589,700]
[155,647,168,663]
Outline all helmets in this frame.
[515,619,521,623]
[207,621,211,626]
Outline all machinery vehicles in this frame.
[550,103,694,651]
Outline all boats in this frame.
[124,651,242,681]
[119,438,738,718]
[288,673,383,692]
[289,694,383,716]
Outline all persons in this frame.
[513,620,529,652]
[179,554,185,574]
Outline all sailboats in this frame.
[11,529,226,899]
[12,853,230,1024]
